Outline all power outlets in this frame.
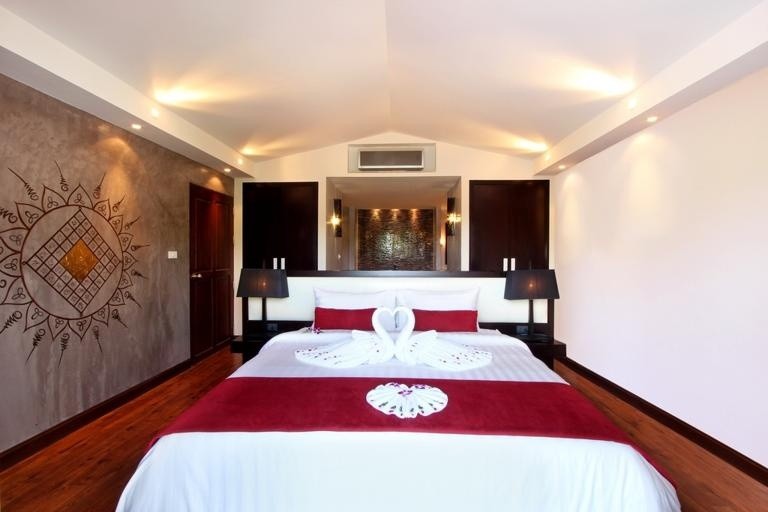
[516,325,529,336]
[266,322,278,332]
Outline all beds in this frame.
[114,270,683,512]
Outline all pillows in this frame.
[410,306,478,333]
[312,286,396,331]
[313,306,377,332]
[394,285,482,332]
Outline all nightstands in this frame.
[241,335,272,364]
[509,333,567,371]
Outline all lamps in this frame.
[236,267,290,334]
[503,269,561,343]
